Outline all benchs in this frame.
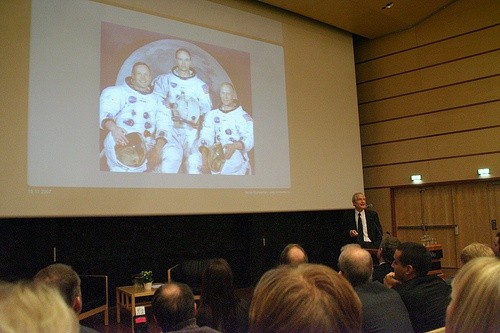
[77,275,109,325]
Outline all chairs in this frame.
[168,264,180,284]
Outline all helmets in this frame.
[114,132,147,167]
[207,143,226,172]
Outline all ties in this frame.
[357,212,365,245]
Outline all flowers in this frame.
[136,270,154,282]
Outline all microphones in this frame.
[372,222,383,239]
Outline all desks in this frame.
[116,283,200,333]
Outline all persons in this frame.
[34,263,99,333]
[493,232,500,257]
[0,280,81,332]
[373,237,401,283]
[250,263,362,333]
[384,242,451,333]
[338,247,414,333]
[197,83,254,175]
[153,48,211,174]
[99,62,173,173]
[152,282,221,333]
[343,193,383,249]
[198,257,253,332]
[445,256,500,333]
[280,243,308,265]
[461,242,495,261]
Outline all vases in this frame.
[144,282,152,290]
